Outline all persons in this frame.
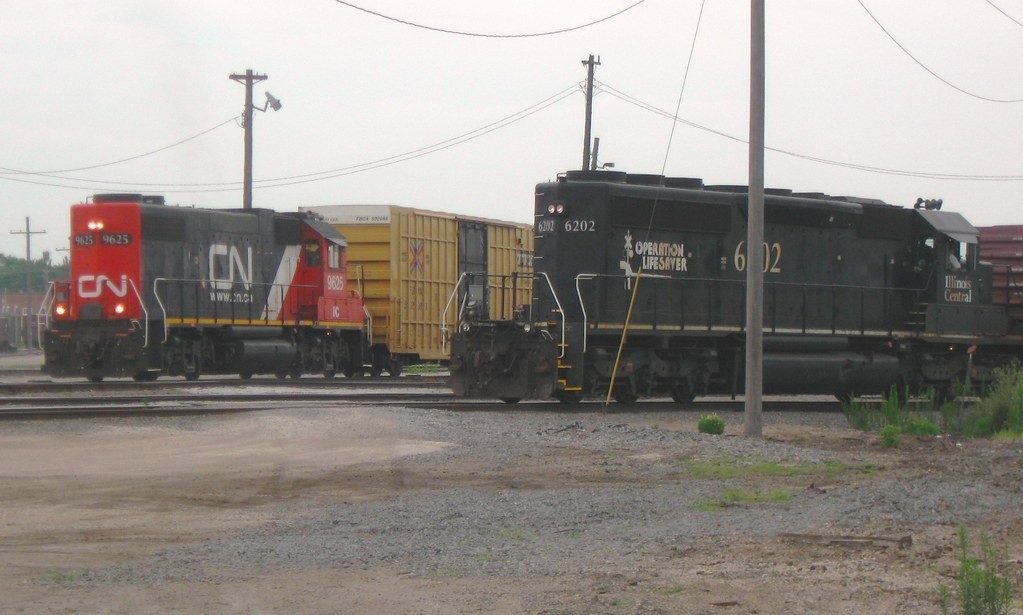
[949,242,962,269]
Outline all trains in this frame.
[32,191,535,383]
[441,169,1023,408]
